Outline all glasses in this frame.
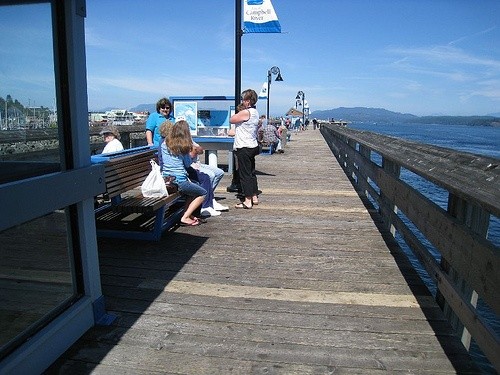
[161,106,170,109]
[103,132,109,135]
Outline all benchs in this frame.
[258,131,278,155]
[91,146,190,241]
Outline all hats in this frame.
[99,128,112,135]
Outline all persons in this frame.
[145,98,176,154]
[159,118,230,228]
[313,118,317,130]
[257,115,309,154]
[229,89,259,209]
[99,126,124,154]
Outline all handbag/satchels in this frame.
[164,175,178,193]
[142,160,170,198]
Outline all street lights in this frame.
[267,66,284,125]
[296,91,304,130]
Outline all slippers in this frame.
[235,202,258,209]
[180,220,201,226]
[192,216,208,223]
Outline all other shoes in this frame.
[227,184,239,191]
[213,203,230,210]
[201,206,222,216]
